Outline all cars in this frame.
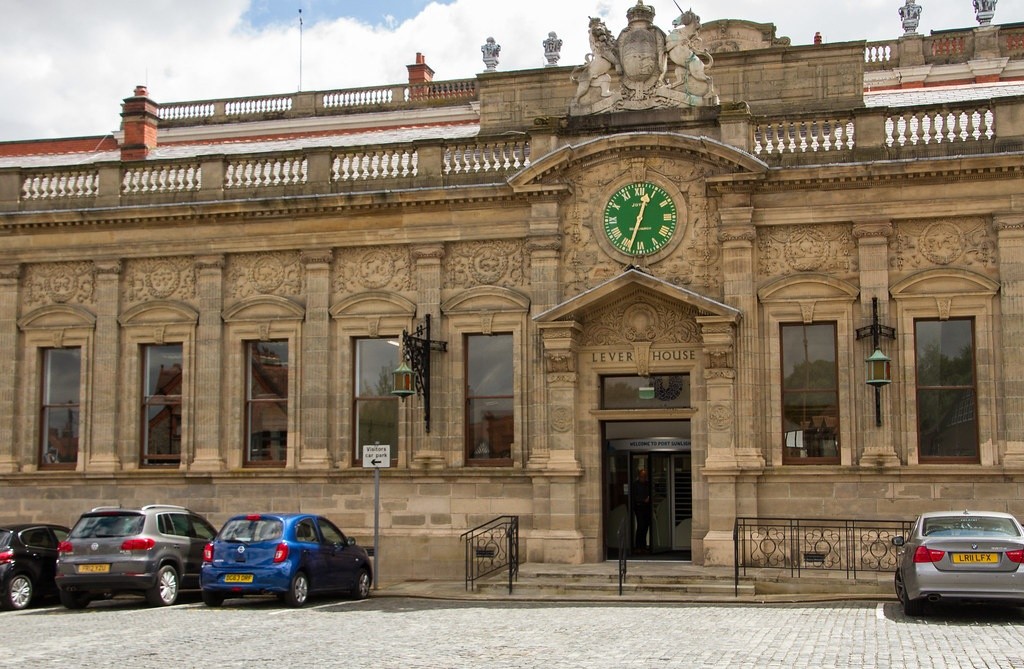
[198,512,374,607]
[0,523,116,610]
[890,510,1024,616]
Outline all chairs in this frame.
[297,524,312,538]
[259,523,279,540]
[29,531,46,547]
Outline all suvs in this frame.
[53,503,220,608]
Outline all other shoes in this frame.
[636,545,651,553]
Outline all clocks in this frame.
[593,166,688,267]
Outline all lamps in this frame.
[391,314,448,434]
[856,297,897,427]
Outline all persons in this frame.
[631,468,653,556]
